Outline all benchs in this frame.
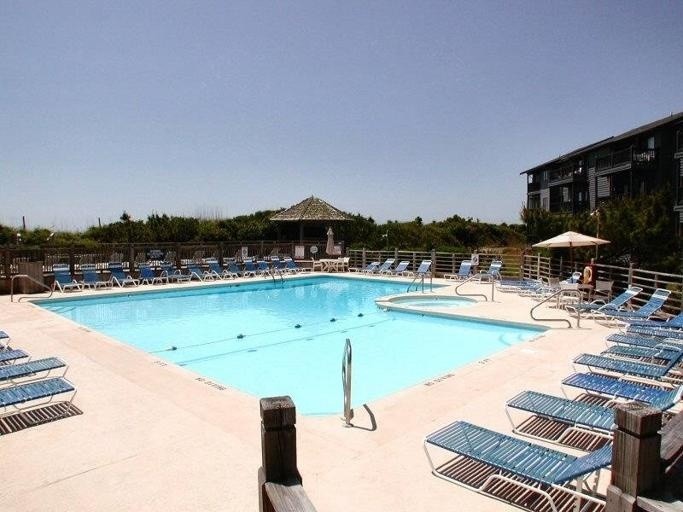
[0,332,78,425]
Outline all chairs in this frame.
[424,418,613,512]
[503,384,682,444]
[496,285,683,398]
[51,253,302,292]
[311,250,582,294]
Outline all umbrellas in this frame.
[325,227,335,260]
[532,231,611,275]
